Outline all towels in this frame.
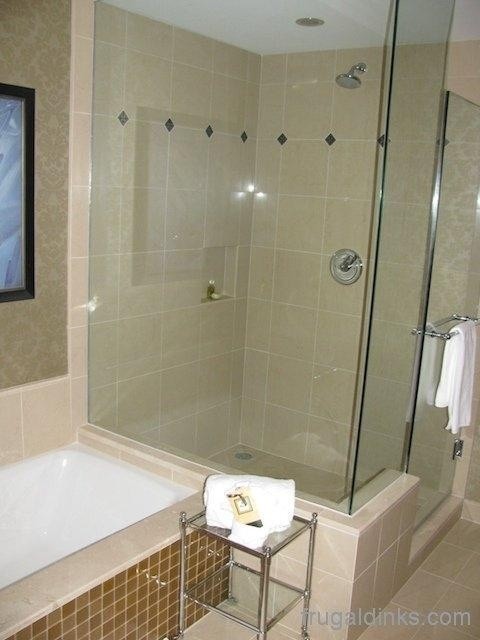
[433,319,479,436]
[202,473,297,550]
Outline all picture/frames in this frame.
[3,81,38,303]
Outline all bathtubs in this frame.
[0,441,200,590]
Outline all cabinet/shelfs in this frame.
[178,505,319,640]
